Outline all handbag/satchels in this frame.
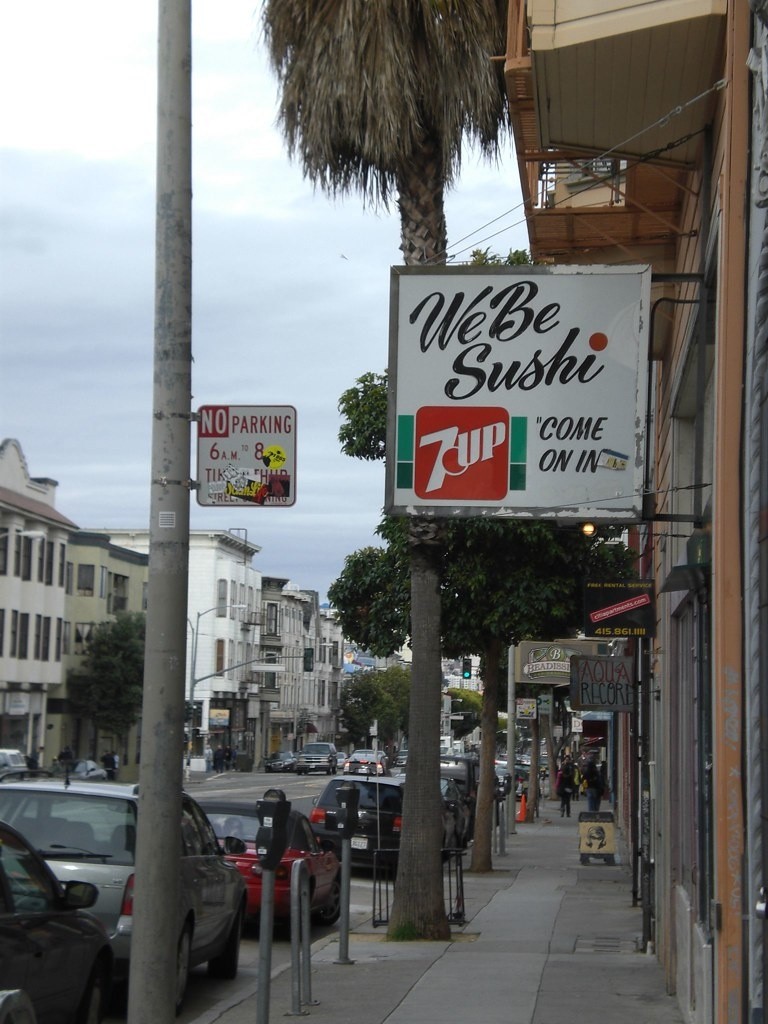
[583,778,588,788]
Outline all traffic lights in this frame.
[462,657,473,681]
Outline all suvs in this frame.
[294,742,338,774]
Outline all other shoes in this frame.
[561,809,565,817]
[567,813,570,817]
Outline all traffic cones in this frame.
[516,792,527,823]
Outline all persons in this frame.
[213,744,239,774]
[555,750,608,817]
[31,746,120,782]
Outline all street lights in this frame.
[185,604,251,772]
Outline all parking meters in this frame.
[336,777,359,965]
[254,789,293,1024]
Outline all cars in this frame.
[307,775,456,875]
[193,790,341,933]
[393,751,551,848]
[394,749,407,765]
[0,777,248,1019]
[336,753,348,769]
[189,797,345,940]
[0,750,27,780]
[262,750,296,773]
[0,820,116,1024]
[54,760,109,784]
[342,749,388,779]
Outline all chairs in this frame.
[110,825,136,852]
[382,795,401,811]
[54,820,94,844]
[213,817,243,838]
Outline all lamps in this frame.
[576,521,598,538]
